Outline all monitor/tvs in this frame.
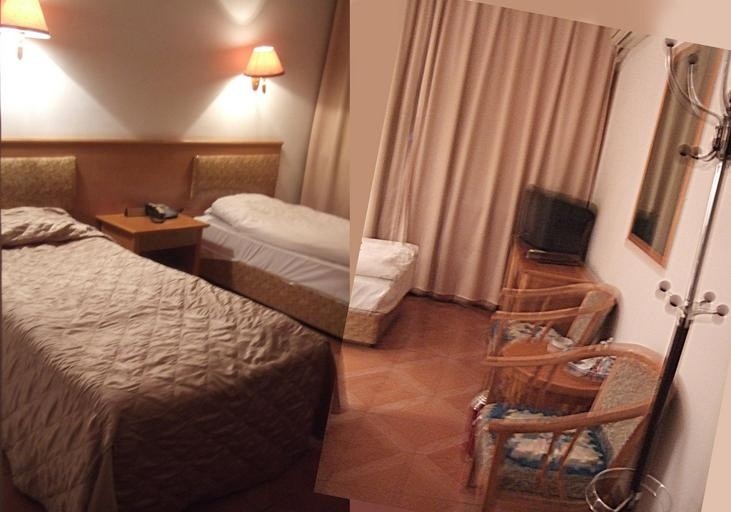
[517,186,596,265]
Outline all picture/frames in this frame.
[626,41,726,268]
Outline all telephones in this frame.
[146,202,178,223]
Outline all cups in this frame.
[547,336,573,355]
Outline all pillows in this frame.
[2,208,112,245]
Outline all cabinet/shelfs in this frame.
[488,235,604,300]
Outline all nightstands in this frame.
[96,208,210,284]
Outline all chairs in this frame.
[466,342,678,512]
[481,282,620,388]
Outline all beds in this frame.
[0,158,350,477]
[180,155,421,347]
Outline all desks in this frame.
[487,336,617,410]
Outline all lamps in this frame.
[241,46,284,95]
[1,1,55,60]
